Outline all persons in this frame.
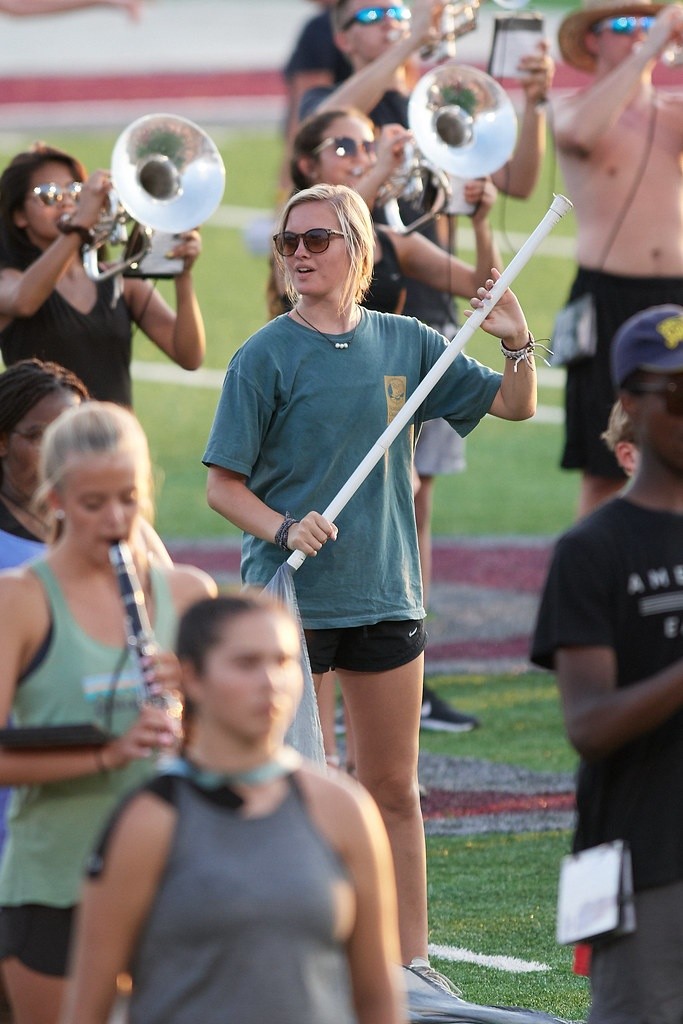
[267,0,554,735]
[66,595,410,1024]
[0,143,205,415]
[0,356,173,1024]
[201,183,539,1002]
[529,305,683,1024]
[549,0,683,518]
[0,401,219,1024]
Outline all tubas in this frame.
[353,65,518,237]
[33,115,228,283]
[382,0,482,69]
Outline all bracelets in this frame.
[500,330,553,373]
[57,221,97,244]
[275,511,299,553]
[95,752,112,778]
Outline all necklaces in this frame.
[0,490,55,534]
[295,304,358,349]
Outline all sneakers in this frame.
[417,682,476,733]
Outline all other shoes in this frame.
[406,956,463,1000]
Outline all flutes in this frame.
[108,536,183,767]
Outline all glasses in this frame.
[639,375,682,416]
[273,226,346,259]
[310,136,377,160]
[10,426,42,445]
[26,181,88,212]
[602,16,653,34]
[341,5,409,32]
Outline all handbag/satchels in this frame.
[0,725,120,750]
[543,295,597,364]
[555,836,637,948]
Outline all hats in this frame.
[610,304,682,387]
[558,0,683,73]
